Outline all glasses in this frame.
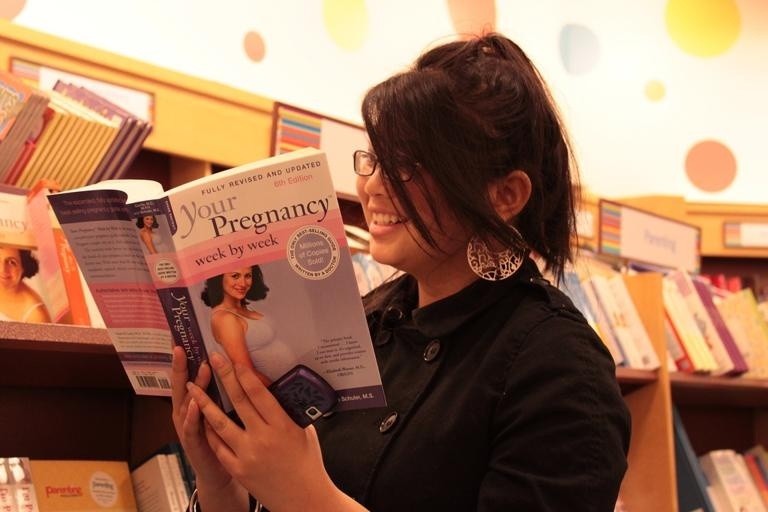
[354,150,423,183]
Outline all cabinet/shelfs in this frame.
[0,20,768,511]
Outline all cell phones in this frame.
[226,364,339,428]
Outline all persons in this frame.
[169,25,633,512]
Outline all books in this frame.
[673,404,768,512]
[542,260,660,371]
[2,71,406,512]
[664,268,768,380]
